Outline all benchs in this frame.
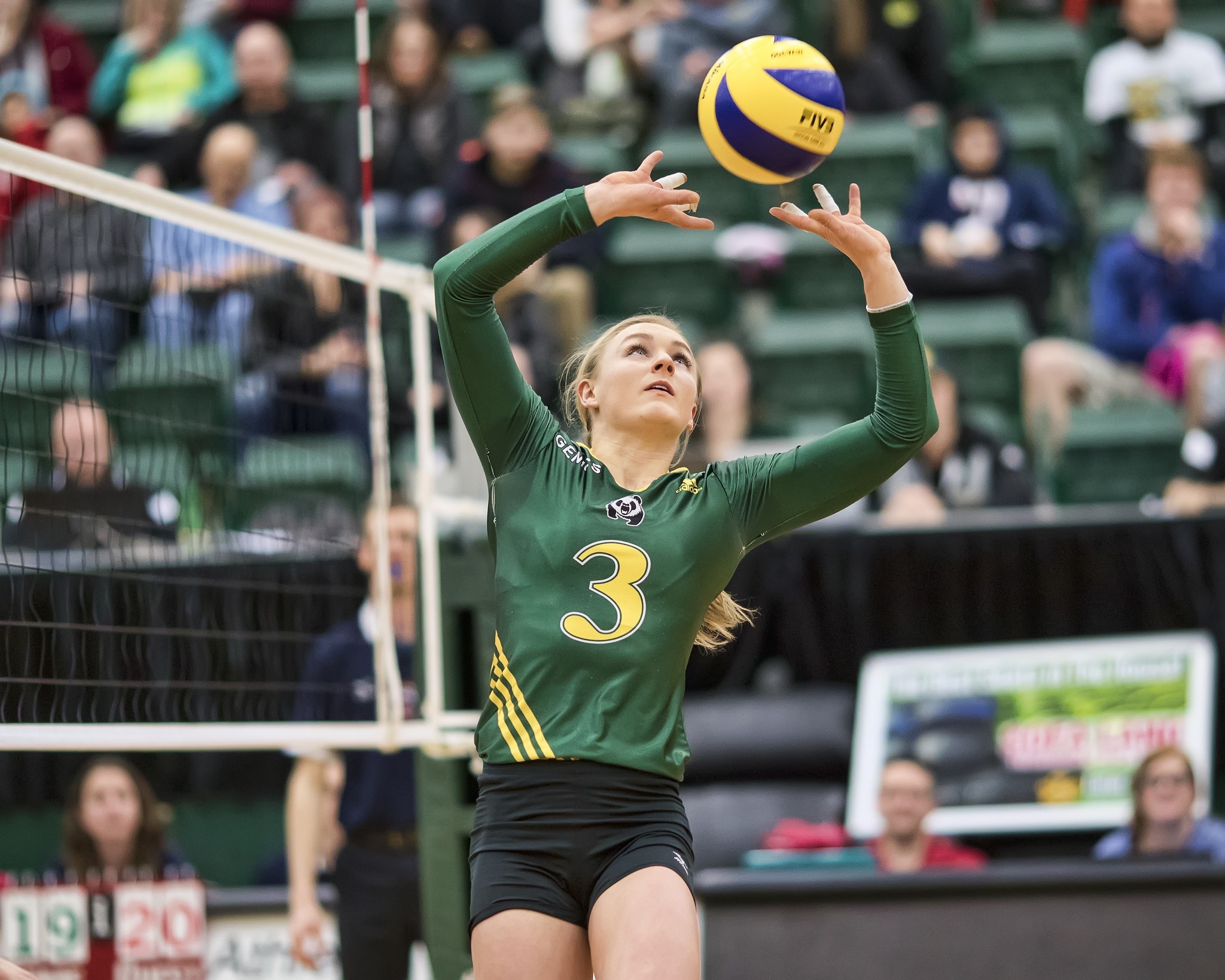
[0,0,1225,510]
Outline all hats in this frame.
[478,79,543,121]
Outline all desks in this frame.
[693,857,1225,980]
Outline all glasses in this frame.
[1145,772,1190,788]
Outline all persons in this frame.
[39,757,196,880]
[0,0,1225,555]
[1086,748,1225,862]
[433,149,940,980]
[863,755,981,873]
[280,496,446,980]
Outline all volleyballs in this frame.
[697,35,846,186]
